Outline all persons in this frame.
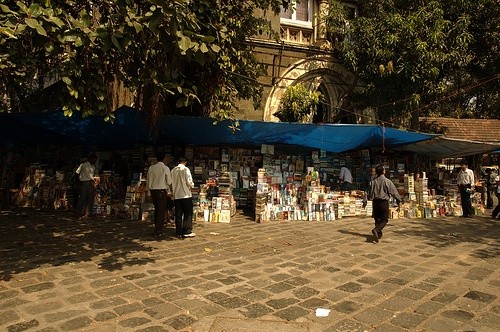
[457,159,475,217]
[339,165,352,191]
[148,152,172,236]
[171,160,196,238]
[362,164,402,242]
[492,181,500,220]
[75,154,98,219]
[485,168,497,209]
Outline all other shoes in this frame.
[372,228,378,242]
[459,215,468,217]
[492,215,495,220]
[152,233,166,237]
[183,233,196,237]
[470,214,472,218]
[378,231,382,239]
[177,234,182,237]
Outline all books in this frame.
[0,150,159,226]
[362,148,500,220]
[164,146,366,224]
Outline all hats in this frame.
[459,160,468,164]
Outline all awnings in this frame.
[0,104,500,161]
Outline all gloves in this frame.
[398,202,402,208]
[362,201,367,208]
